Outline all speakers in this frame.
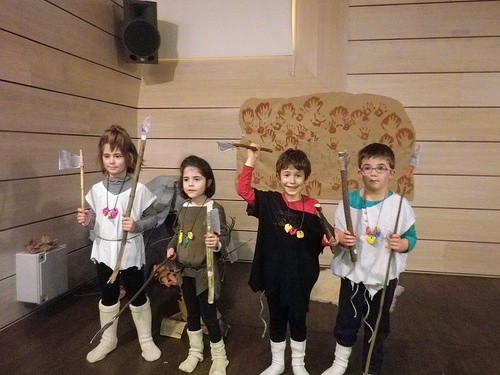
[121,0,161,64]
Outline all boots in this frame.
[260,339,287,375]
[179,327,204,372]
[128,294,162,362]
[320,340,353,375]
[86,298,121,363]
[208,336,230,375]
[290,337,310,375]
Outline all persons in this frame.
[167,155,229,375]
[76,124,162,364]
[321,143,417,375]
[237,143,340,375]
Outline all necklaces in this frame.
[178,197,208,247]
[101,173,127,219]
[284,195,305,238]
[363,190,387,245]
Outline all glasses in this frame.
[357,166,392,175]
